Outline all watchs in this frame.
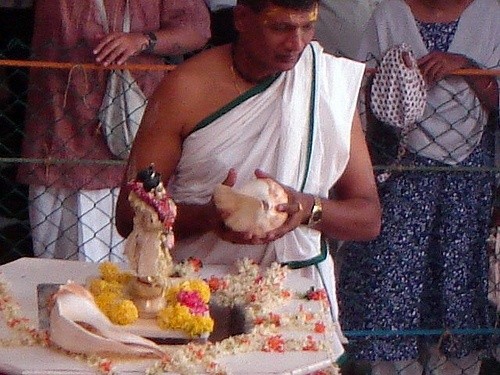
[143,29,158,53]
[308,196,323,227]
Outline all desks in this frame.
[0,258,344,375]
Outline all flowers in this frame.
[1,263,332,373]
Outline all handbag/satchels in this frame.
[94,62,151,158]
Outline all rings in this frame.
[293,201,302,213]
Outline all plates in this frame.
[0,257,334,375]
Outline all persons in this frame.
[116,0,383,375]
[16,1,212,262]
[317,0,500,374]
